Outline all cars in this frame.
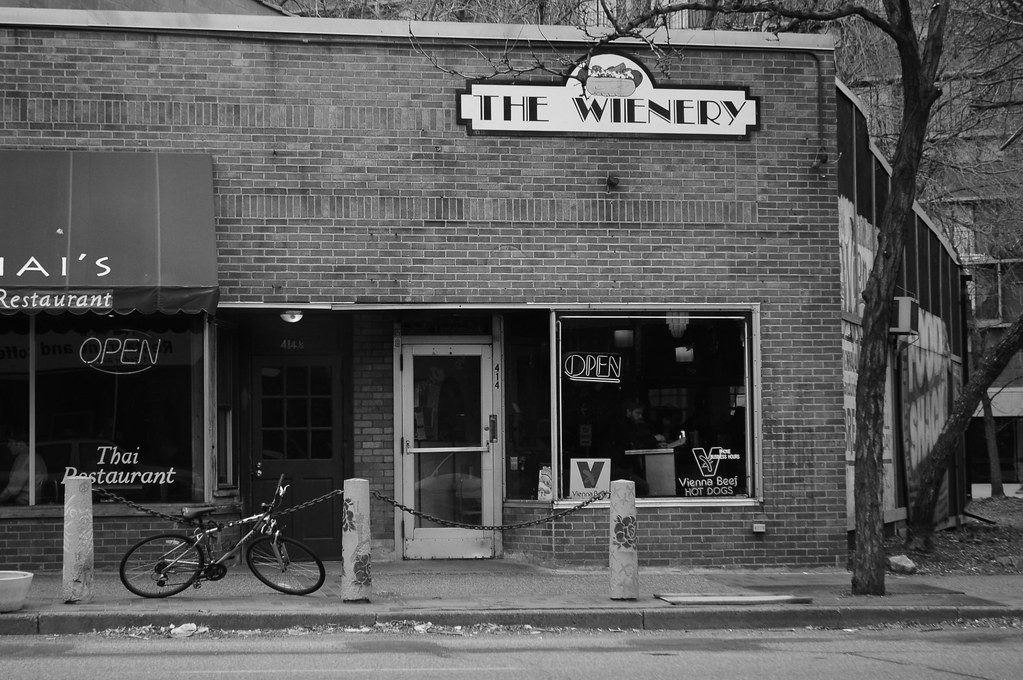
[415,452,483,501]
[1,439,200,509]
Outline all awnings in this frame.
[0,149,221,315]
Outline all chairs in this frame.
[158,479,169,502]
[43,478,59,504]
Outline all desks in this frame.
[625,449,679,497]
[91,484,144,501]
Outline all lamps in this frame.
[676,346,694,365]
[666,312,690,337]
[279,309,302,323]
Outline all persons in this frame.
[0,430,49,506]
[609,403,666,495]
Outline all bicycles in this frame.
[119,474,326,598]
